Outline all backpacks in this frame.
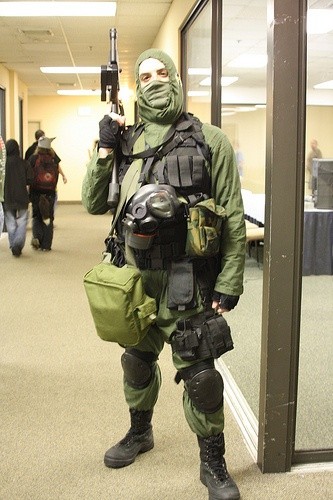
[31,153,58,190]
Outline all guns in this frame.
[101,26,122,208]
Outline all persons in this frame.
[2,139,34,257]
[28,137,58,251]
[83,48,247,500]
[25,129,67,199]
[307,140,322,174]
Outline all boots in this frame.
[196,429,241,500]
[103,408,154,468]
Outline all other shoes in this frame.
[32,239,41,247]
[12,246,21,257]
[40,247,51,252]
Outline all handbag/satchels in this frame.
[84,258,157,346]
[186,197,227,259]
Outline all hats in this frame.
[37,136,56,148]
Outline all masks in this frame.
[140,80,174,109]
